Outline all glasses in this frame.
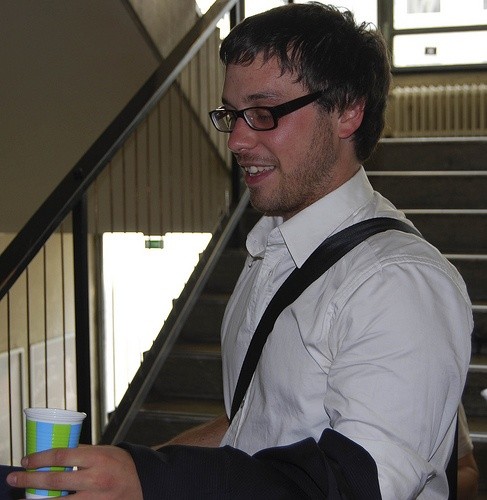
[208,89,331,133]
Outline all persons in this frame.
[6,2,474,499]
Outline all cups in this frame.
[24,408,87,500]
